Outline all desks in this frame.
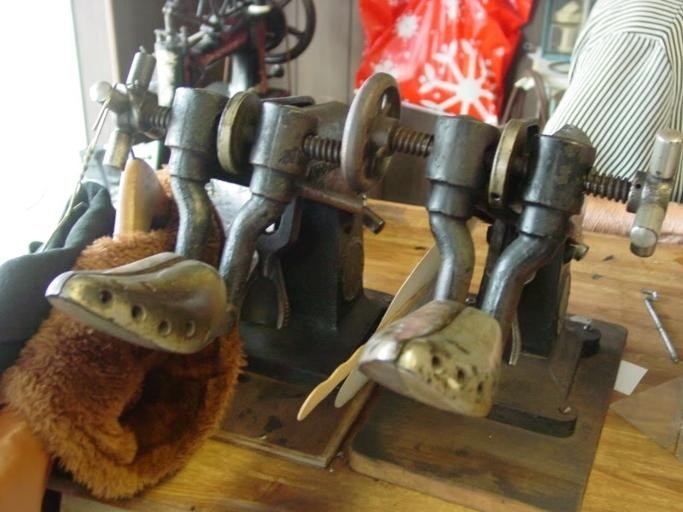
[44,171,681,512]
